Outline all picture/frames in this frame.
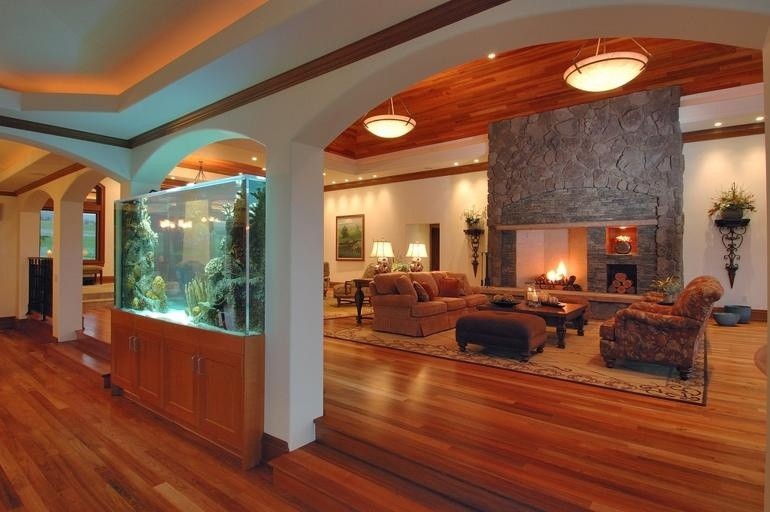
[335,214,366,261]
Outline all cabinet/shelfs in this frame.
[166,323,265,460]
[111,310,164,410]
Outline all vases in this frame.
[720,209,743,220]
[468,222,480,230]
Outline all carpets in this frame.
[323,321,708,407]
[324,287,374,318]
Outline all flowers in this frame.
[462,209,485,226]
[708,180,757,217]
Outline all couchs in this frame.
[599,275,724,381]
[369,270,492,337]
[333,264,378,307]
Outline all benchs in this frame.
[456,309,549,364]
[552,294,591,325]
[83,264,103,284]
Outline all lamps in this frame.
[363,94,417,140]
[561,40,651,93]
[368,239,395,272]
[405,243,429,272]
